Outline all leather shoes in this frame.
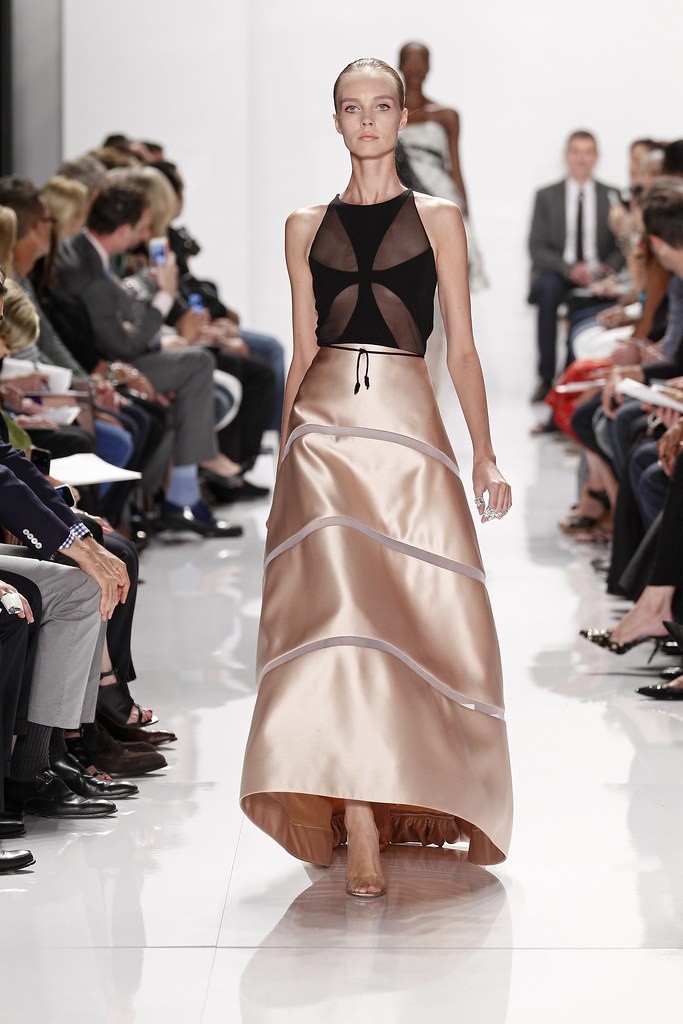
[164,500,243,540]
[14,768,117,818]
[109,727,177,746]
[0,849,36,874]
[0,814,27,839]
[80,739,169,778]
[53,754,139,798]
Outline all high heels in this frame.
[346,823,389,898]
[637,680,683,701]
[559,487,609,531]
[578,623,668,664]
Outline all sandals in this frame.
[97,667,159,729]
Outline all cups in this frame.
[149,235,167,265]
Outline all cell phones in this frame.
[189,294,203,313]
[55,486,75,508]
[148,236,168,276]
[30,448,50,476]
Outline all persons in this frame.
[527,132,683,701]
[240,58,511,899]
[399,42,495,394]
[0,135,284,878]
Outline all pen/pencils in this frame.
[35,366,49,389]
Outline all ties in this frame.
[576,191,585,265]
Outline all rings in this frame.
[474,497,485,507]
[484,507,503,521]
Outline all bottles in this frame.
[188,293,203,315]
